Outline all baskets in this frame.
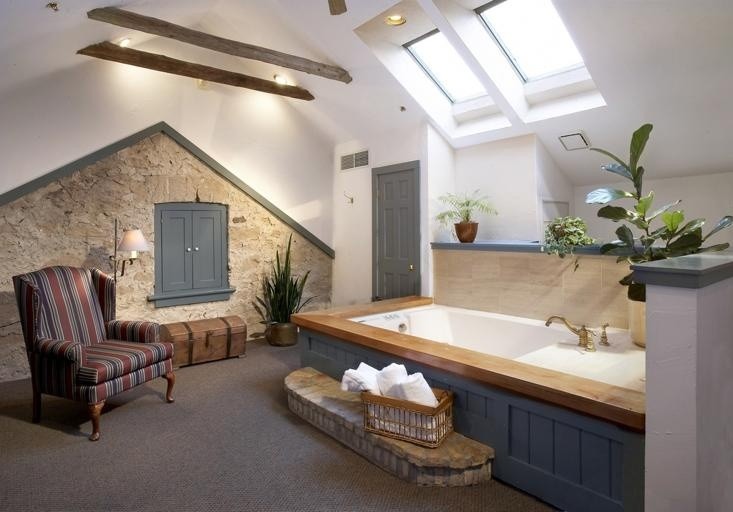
[361,384,456,451]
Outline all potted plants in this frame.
[434,189,500,245]
[584,120,733,350]
[539,216,601,272]
[253,231,320,348]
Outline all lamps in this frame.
[111,228,150,280]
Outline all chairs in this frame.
[11,263,177,443]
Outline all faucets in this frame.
[544,314,596,350]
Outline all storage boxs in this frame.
[160,314,247,370]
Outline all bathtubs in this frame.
[344,302,645,391]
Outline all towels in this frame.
[340,361,450,446]
